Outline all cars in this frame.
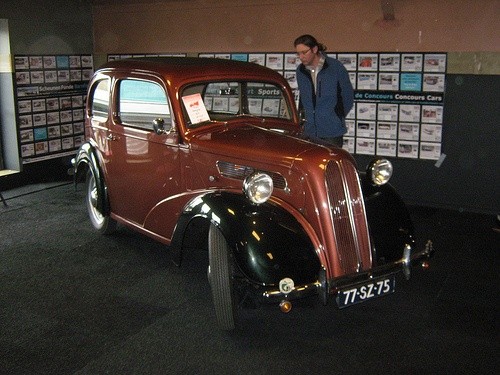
[265,53,442,160]
[15,55,93,155]
[73,57,438,333]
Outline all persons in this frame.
[294,34,354,149]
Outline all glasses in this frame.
[296,48,311,58]
